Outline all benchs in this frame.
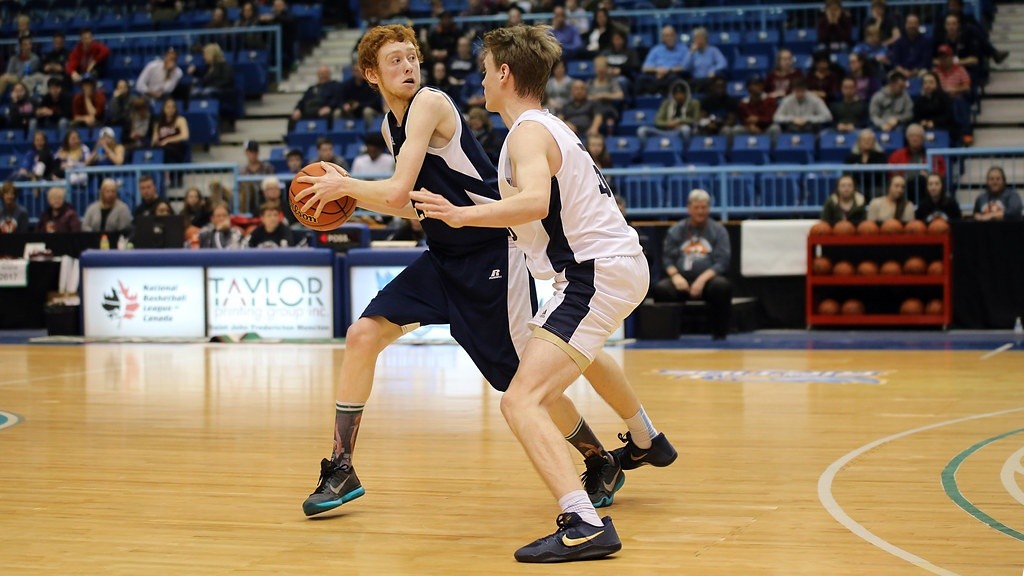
[641,295,759,333]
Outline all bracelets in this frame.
[670,271,679,279]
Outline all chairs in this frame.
[0,0,996,234]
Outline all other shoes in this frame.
[995,50,1009,64]
[962,135,973,145]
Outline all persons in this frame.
[408,25,678,563]
[292,24,625,516]
[0,0,1024,342]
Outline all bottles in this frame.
[99,146,104,157]
[100,235,109,249]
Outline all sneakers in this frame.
[606,431,678,470]
[578,451,626,508]
[302,458,365,516]
[514,512,622,563]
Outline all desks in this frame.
[345,248,463,345]
[82,248,341,348]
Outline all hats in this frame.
[82,72,96,82]
[937,45,953,56]
[244,140,258,152]
[99,127,115,139]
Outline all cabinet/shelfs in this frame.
[804,224,950,334]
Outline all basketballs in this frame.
[289,161,356,232]
